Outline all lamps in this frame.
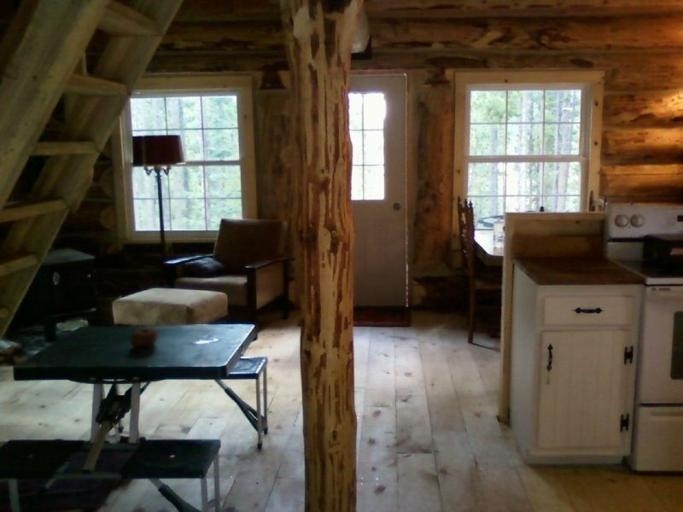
[131,136,182,257]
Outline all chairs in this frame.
[458,195,503,343]
[162,218,297,341]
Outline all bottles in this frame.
[493,216,504,249]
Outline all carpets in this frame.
[298,306,411,328]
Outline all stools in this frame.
[110,287,230,324]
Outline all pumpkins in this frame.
[130,328,155,348]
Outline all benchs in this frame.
[2,439,221,512]
[215,356,268,449]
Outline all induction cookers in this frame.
[618,257,683,284]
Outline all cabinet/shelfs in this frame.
[509,265,643,467]
[95,238,217,322]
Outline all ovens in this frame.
[636,283,683,475]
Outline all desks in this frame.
[13,324,258,512]
[474,229,504,268]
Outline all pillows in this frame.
[182,257,235,277]
